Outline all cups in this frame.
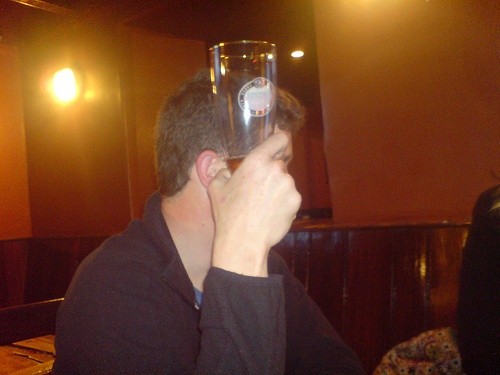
[210,42,277,178]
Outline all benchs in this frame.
[273,219,471,375]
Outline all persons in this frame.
[48,69,366,374]
[453,183,500,374]
